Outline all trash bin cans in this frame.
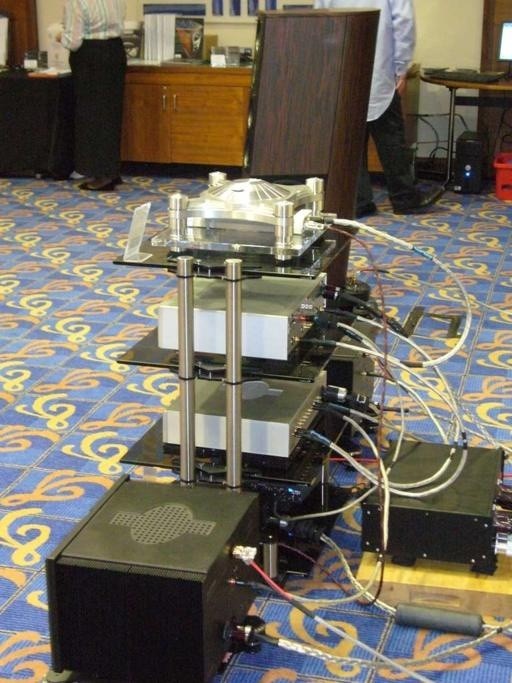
[493,151,512,199]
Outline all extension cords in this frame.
[415,166,446,182]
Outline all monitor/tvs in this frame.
[495,21,512,78]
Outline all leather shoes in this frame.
[394,186,445,214]
[81,182,114,191]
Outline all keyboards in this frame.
[430,71,499,84]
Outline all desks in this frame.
[119,65,254,167]
[415,71,512,190]
[369,63,420,173]
[0,71,74,177]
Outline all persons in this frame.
[48,0,126,179]
[314,0,444,214]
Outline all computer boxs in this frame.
[453,131,488,194]
